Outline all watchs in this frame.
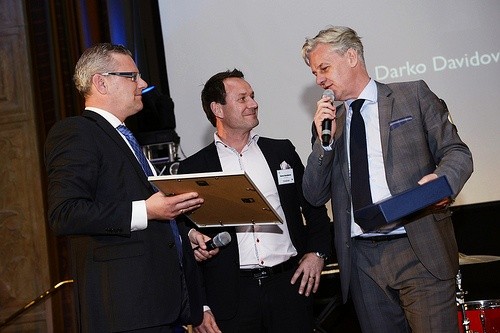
[316,252,327,260]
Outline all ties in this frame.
[116,124,182,266]
[349,99,372,233]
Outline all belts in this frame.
[352,233,408,243]
[240,253,304,279]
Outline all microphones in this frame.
[184,232,232,263]
[321,89,335,147]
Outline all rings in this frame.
[180,209,183,214]
[309,276,314,279]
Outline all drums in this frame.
[457,299,500,333]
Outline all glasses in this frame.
[91,71,141,82]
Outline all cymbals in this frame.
[458,253,500,265]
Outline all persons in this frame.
[178,68,332,333]
[45,43,218,333]
[302,27,473,333]
